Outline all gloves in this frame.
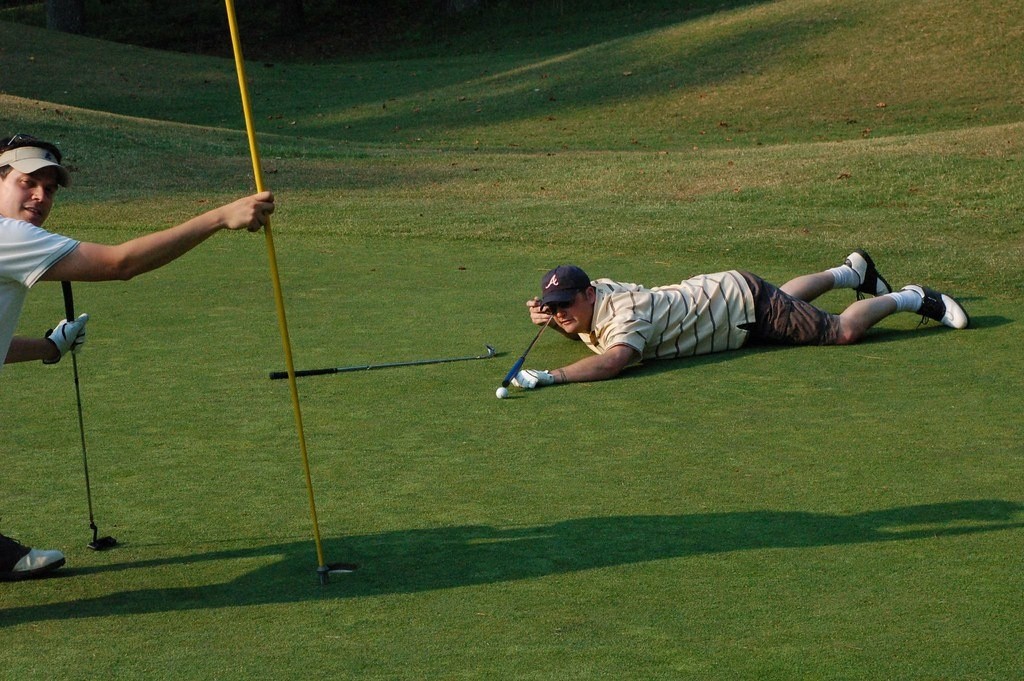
[511,370,554,389]
[43,313,89,364]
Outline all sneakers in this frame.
[900,284,970,332]
[0,533,66,580]
[844,248,892,301]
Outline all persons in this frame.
[0,134,275,579]
[510,247,970,388]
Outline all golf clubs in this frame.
[61,280,117,551]
[268,344,497,380]
[502,315,553,387]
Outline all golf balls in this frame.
[495,386,508,399]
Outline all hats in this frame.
[540,265,590,312]
[0,146,72,187]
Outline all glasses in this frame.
[0,133,37,156]
[545,287,588,315]
